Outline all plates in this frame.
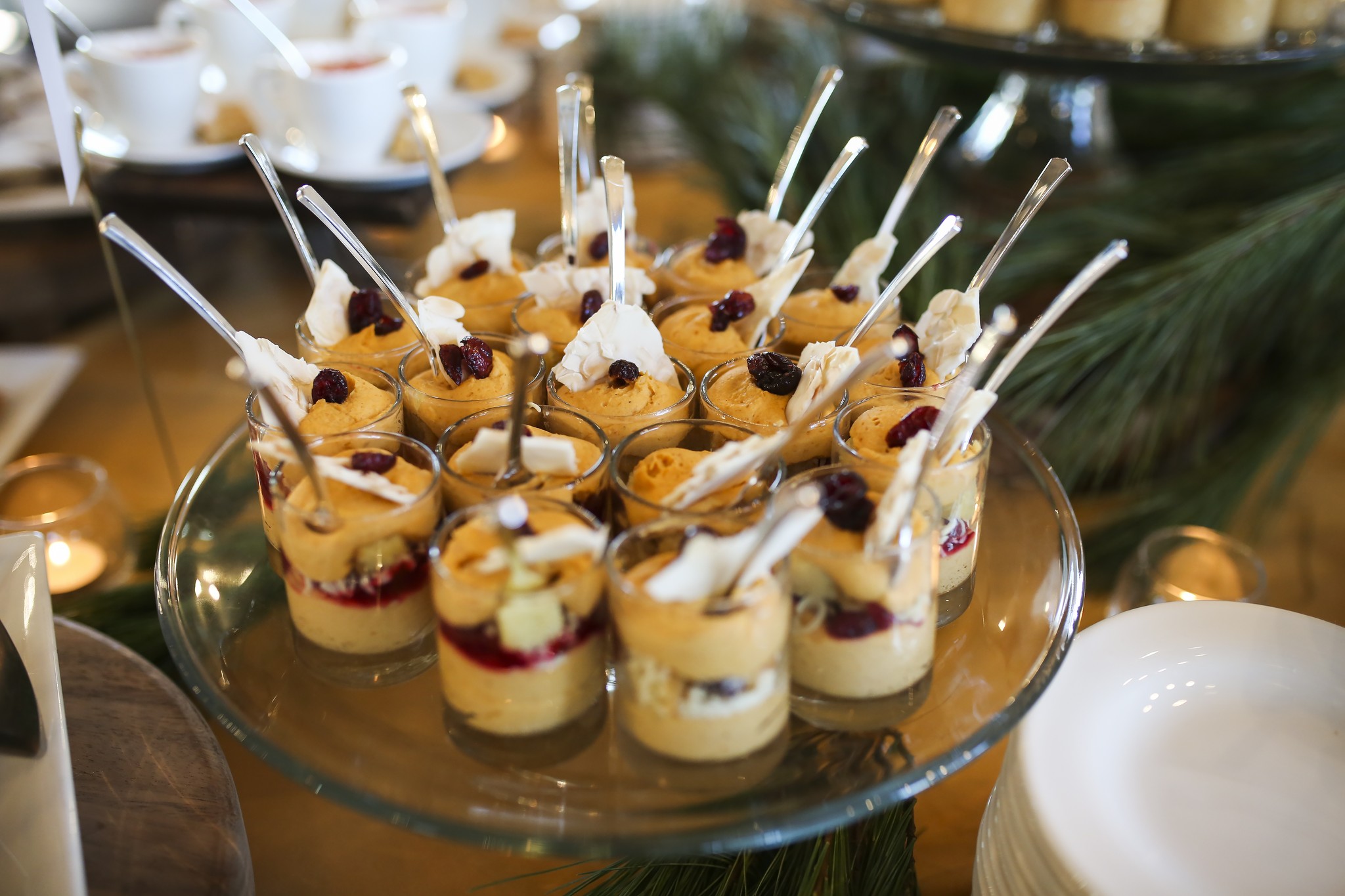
[0,532,88,896]
[451,1,560,111]
[970,599,1345,896]
[81,112,508,190]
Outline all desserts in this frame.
[232,170,999,759]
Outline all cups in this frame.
[245,239,992,762]
[78,2,461,161]
[0,453,137,611]
[876,1,1343,47]
[1106,524,1266,620]
